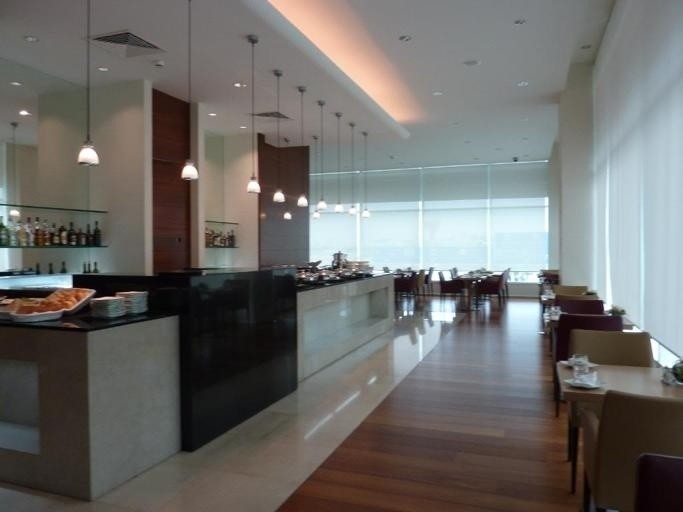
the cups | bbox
[572,354,589,381]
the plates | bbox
[90,296,126,320]
[561,359,600,370]
[117,292,148,315]
[564,378,606,389]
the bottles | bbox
[86,223,94,246]
[36,261,99,274]
[78,227,88,246]
[0,215,50,248]
[93,220,101,246]
[68,222,78,246]
[205,227,235,248]
[58,226,68,245]
[50,222,61,245]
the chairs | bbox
[539,268,682,512]
[382,267,510,311]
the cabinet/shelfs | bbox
[0,202,109,276]
